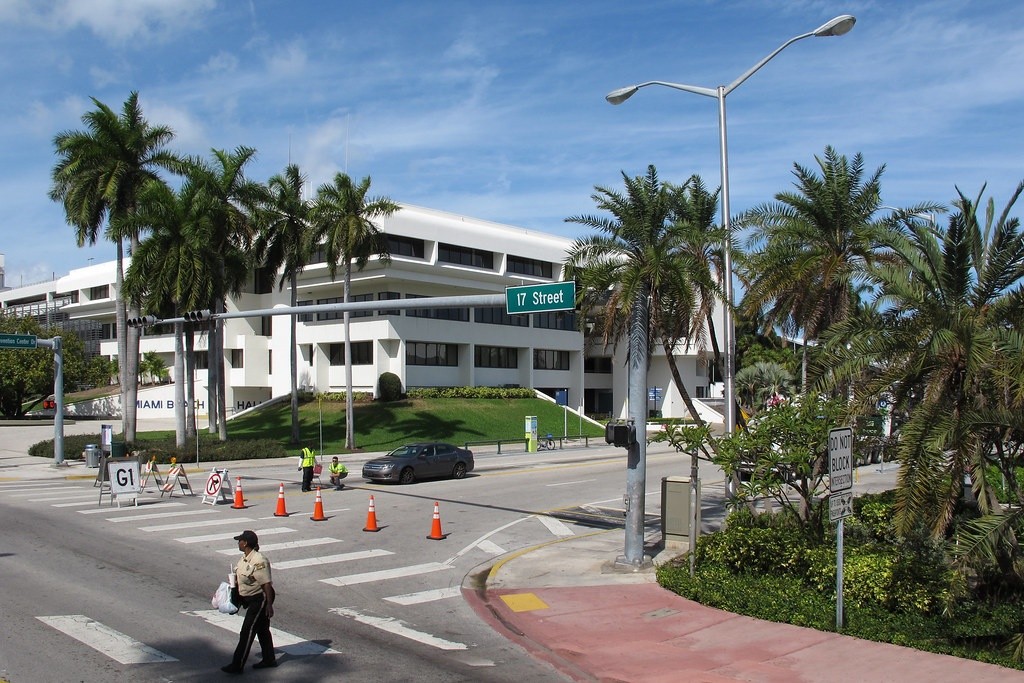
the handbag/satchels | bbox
[211,582,238,613]
[314,465,321,473]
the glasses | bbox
[333,460,338,462]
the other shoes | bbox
[340,484,344,489]
[333,485,341,490]
[252,658,278,669]
[302,487,311,492]
[221,662,244,674]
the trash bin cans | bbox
[85,444,98,468]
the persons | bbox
[220,530,279,675]
[297,442,318,492]
[329,457,349,490]
[767,391,781,408]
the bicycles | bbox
[537,430,556,451]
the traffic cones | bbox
[274,482,289,517]
[310,486,328,521]
[231,476,248,509]
[425,501,447,540]
[362,494,381,532]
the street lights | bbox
[605,11,859,486]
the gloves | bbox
[298,467,302,471]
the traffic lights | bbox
[126,314,156,329]
[183,310,210,323]
[603,422,629,446]
[43,401,55,409]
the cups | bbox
[228,573,236,588]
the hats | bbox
[234,530,258,546]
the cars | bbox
[361,442,474,486]
